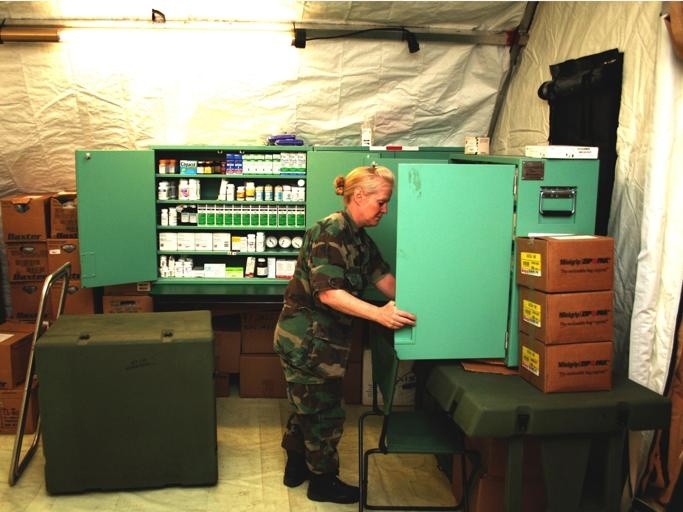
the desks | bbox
[414,360,670,510]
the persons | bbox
[274,166,417,505]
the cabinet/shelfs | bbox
[378,154,600,369]
[78,147,469,301]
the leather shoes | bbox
[307,474,359,504]
[283,451,308,488]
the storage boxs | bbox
[242,315,281,352]
[239,353,289,397]
[48,278,96,319]
[102,284,152,295]
[511,232,617,292]
[11,281,51,320]
[6,242,51,284]
[515,332,616,395]
[215,329,239,375]
[216,371,229,398]
[1,320,46,340]
[0,379,40,436]
[1,332,32,387]
[52,191,79,237]
[2,193,59,242]
[514,290,617,345]
[102,296,153,312]
[48,236,82,278]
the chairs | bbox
[356,348,470,512]
